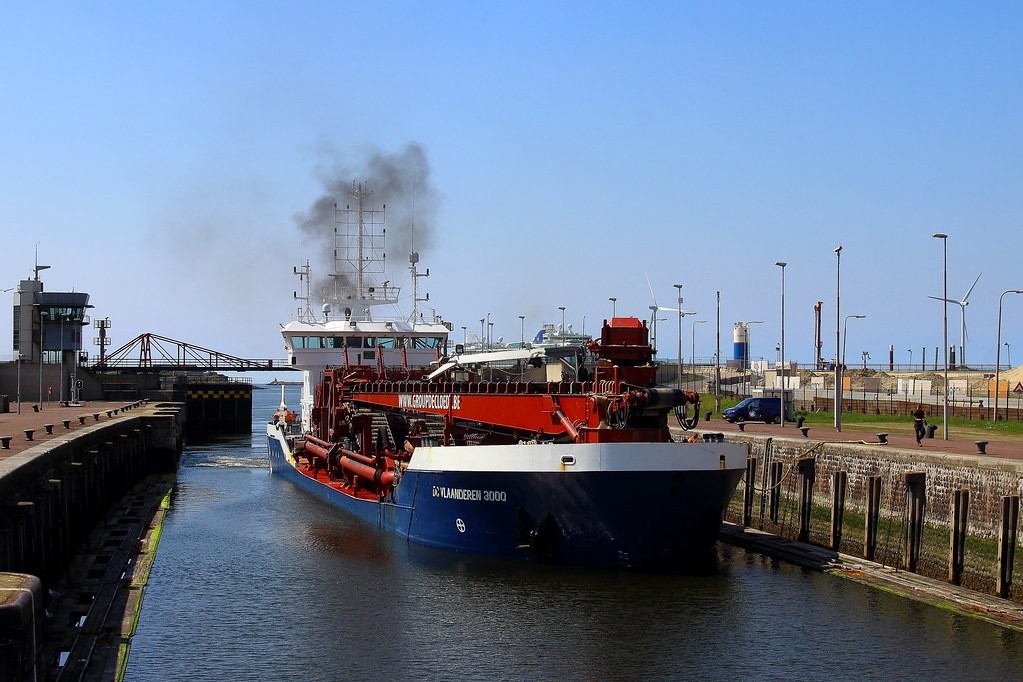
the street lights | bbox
[932,233,948,440]
[518,316,525,350]
[644,272,697,362]
[673,284,683,391]
[481,312,494,353]
[1003,343,1010,369]
[558,306,565,348]
[774,262,788,426]
[692,320,707,393]
[40,312,48,410]
[841,315,866,413]
[928,273,982,371]
[461,326,466,355]
[609,298,618,319]
[833,246,841,432]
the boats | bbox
[267,179,748,560]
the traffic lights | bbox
[75,380,83,389]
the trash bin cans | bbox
[0,395,9,413]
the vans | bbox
[722,396,789,424]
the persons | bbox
[914,404,928,447]
